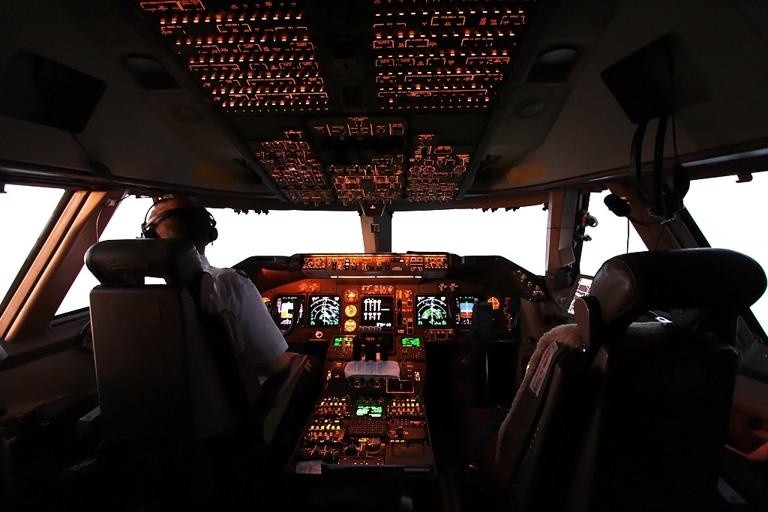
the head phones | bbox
[629,110,689,221]
[143,207,217,242]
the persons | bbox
[147,200,300,377]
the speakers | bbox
[600,32,707,126]
[0,48,106,135]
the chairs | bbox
[489,248,768,509]
[76,237,325,471]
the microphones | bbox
[604,194,669,226]
